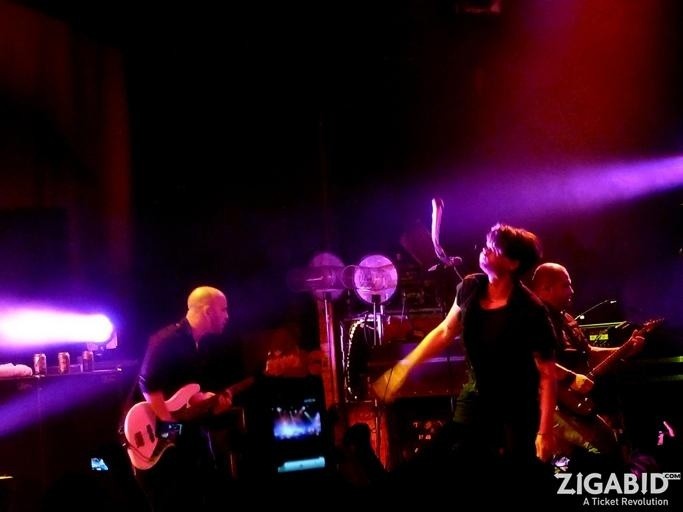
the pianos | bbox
[338,306,475,473]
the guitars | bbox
[123,378,254,471]
[556,318,665,421]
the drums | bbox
[352,255,399,305]
[304,253,346,301]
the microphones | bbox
[427,256,462,272]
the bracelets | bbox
[535,428,555,437]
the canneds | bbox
[59,352,70,374]
[82,350,93,372]
[33,354,48,375]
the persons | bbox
[368,221,563,510]
[117,283,258,510]
[529,262,649,511]
